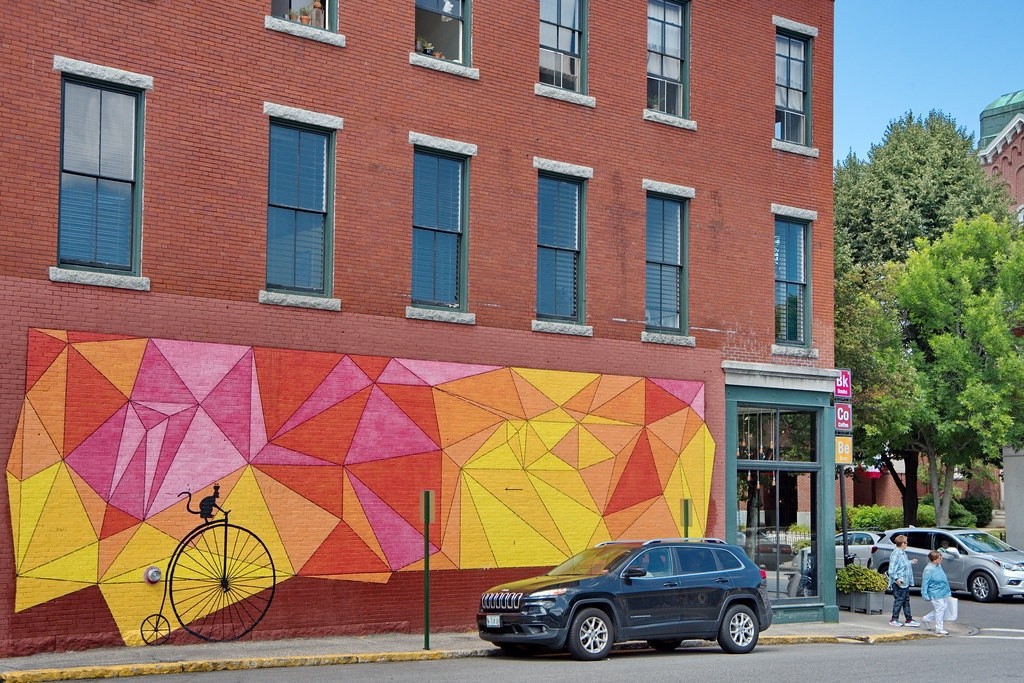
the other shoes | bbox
[904,620,920,626]
[935,629,949,635]
[889,620,904,627]
[921,616,932,631]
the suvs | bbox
[475,537,775,660]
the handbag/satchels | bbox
[943,597,959,621]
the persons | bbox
[937,538,951,553]
[920,550,952,635]
[888,535,921,627]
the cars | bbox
[736,532,795,570]
[870,525,1024,602]
[796,531,887,570]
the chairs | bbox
[647,553,664,572]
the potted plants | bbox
[652,94,658,110]
[836,563,888,615]
[288,9,298,21]
[432,49,443,58]
[423,43,434,55]
[416,33,428,53]
[299,6,312,25]
